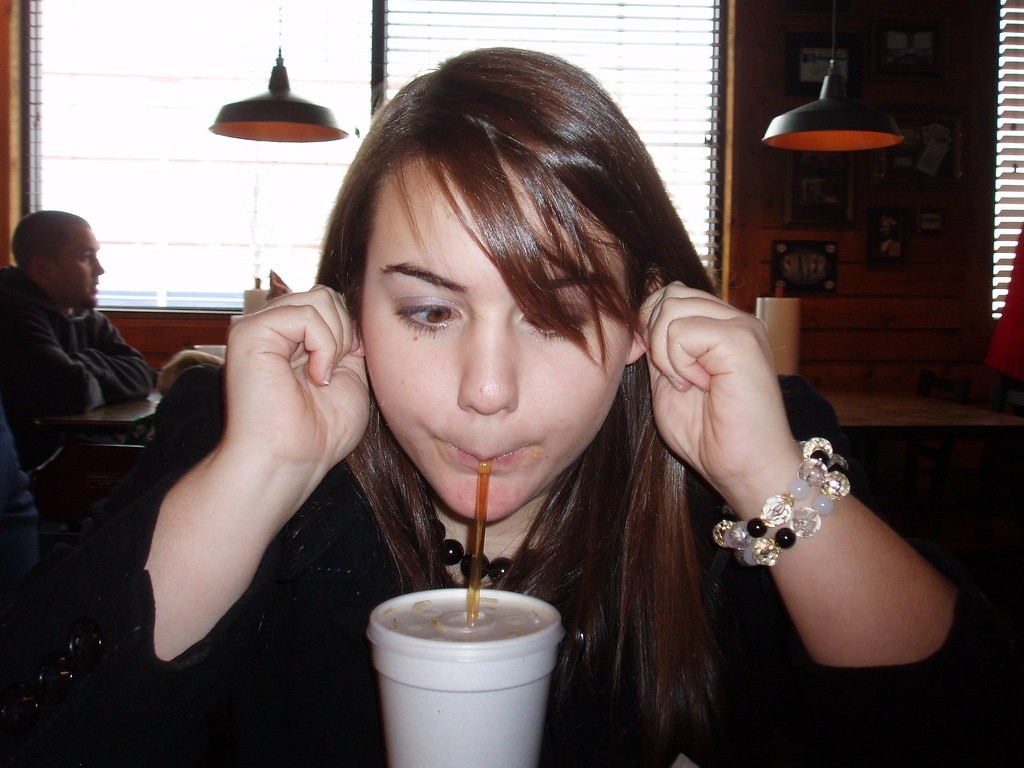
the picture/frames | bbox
[877,105,966,184]
[916,209,946,235]
[785,30,866,100]
[868,12,950,82]
[865,204,914,264]
[780,148,857,227]
[770,238,837,295]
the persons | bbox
[0,48,1024,768]
[0,212,227,709]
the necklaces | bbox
[423,501,545,590]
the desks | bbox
[810,388,1023,526]
[26,386,166,480]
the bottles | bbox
[243,277,268,315]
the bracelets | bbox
[712,437,852,567]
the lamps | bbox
[763,1,906,150]
[209,0,350,142]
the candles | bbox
[755,297,801,377]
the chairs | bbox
[975,373,1024,513]
[863,370,973,507]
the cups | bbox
[195,344,226,361]
[364,587,565,768]
[756,297,800,380]
